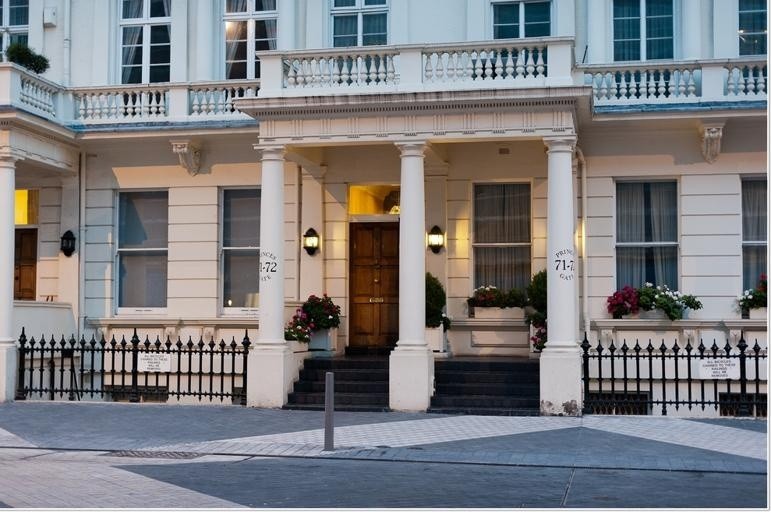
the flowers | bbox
[468,285,528,307]
[287,292,341,336]
[605,282,690,320]
[737,273,769,308]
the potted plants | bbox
[425,271,450,352]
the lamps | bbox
[59,230,76,255]
[303,230,319,255]
[427,225,444,255]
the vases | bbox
[473,305,526,319]
[620,306,669,321]
[308,325,339,351]
[748,306,767,321]
